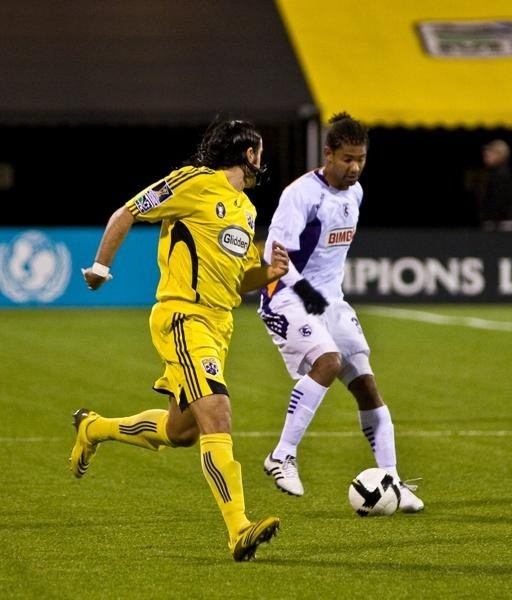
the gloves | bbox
[290,278,330,315]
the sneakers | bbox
[263,452,304,497]
[227,516,280,562]
[399,477,425,512]
[68,407,101,479]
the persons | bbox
[472,140,512,305]
[263,112,424,513]
[69,118,289,562]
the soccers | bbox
[348,468,401,517]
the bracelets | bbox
[92,262,112,281]
